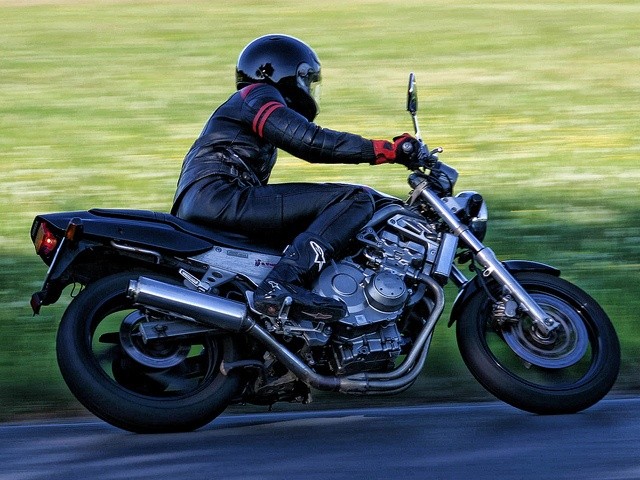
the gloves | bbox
[371,132,420,166]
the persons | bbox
[170,34,420,321]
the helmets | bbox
[235,34,322,122]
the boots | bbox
[253,231,347,323]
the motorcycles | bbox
[30,74,620,433]
